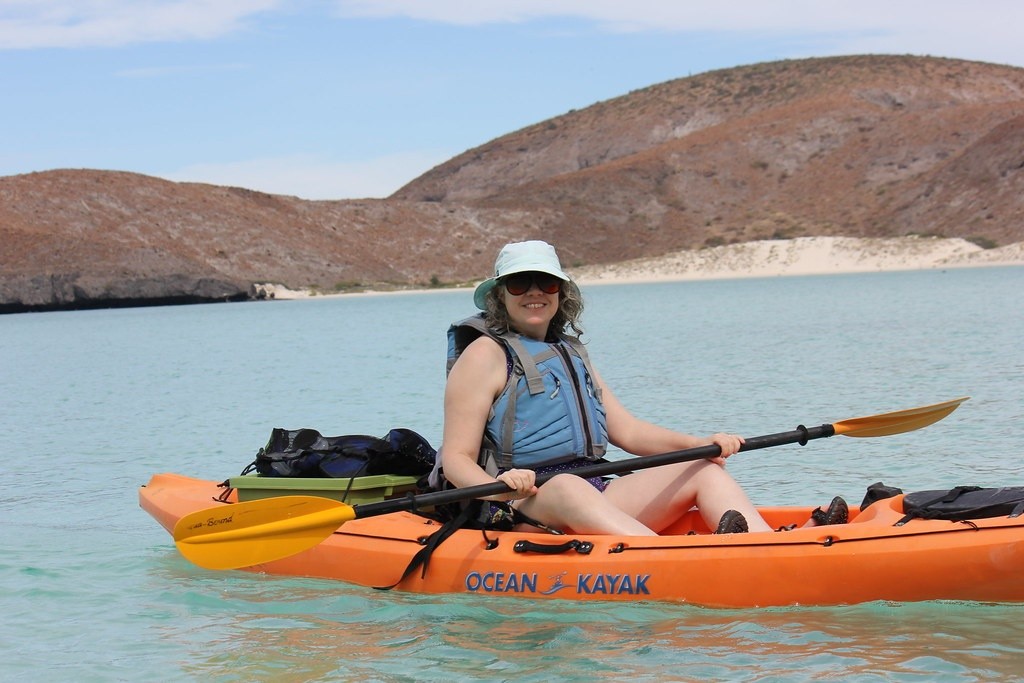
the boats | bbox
[135,463,1023,611]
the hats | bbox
[474,240,571,310]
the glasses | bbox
[499,271,563,296]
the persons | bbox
[442,239,850,538]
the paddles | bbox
[172,395,975,571]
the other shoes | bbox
[685,509,748,535]
[774,496,848,532]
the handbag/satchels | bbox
[212,428,436,504]
